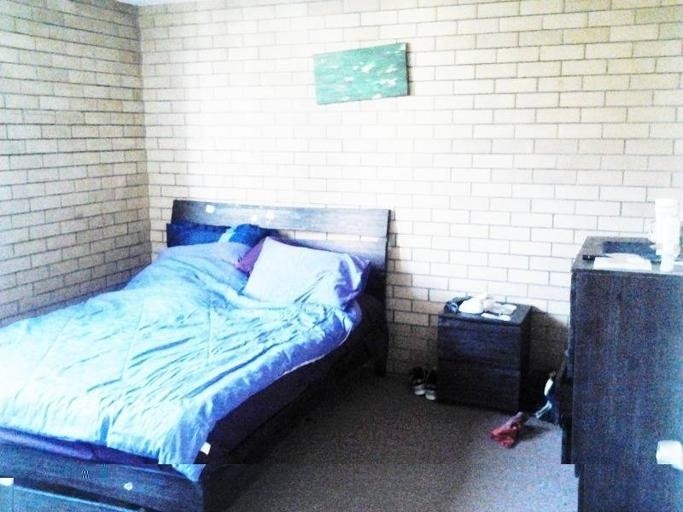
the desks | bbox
[436,302,531,414]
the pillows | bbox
[161,218,372,316]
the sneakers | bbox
[413,366,437,400]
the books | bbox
[601,239,662,265]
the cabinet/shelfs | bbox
[571,236,683,512]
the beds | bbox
[0,201,390,511]
